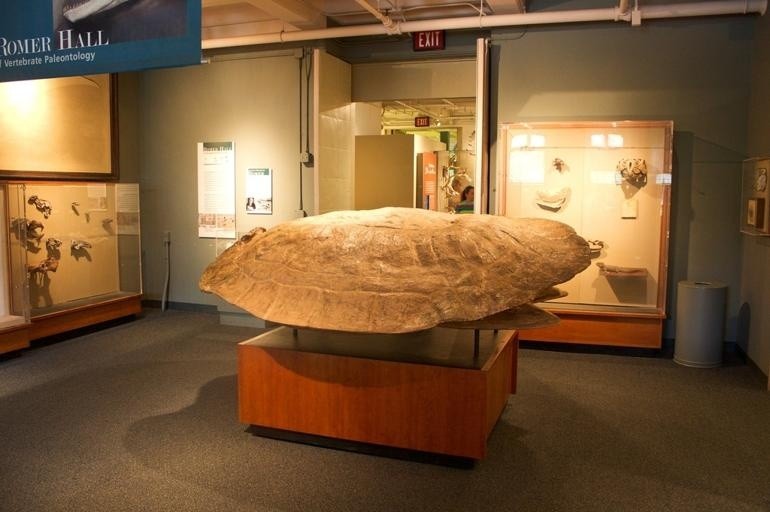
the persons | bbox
[455,186,474,213]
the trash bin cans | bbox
[671,281,728,370]
